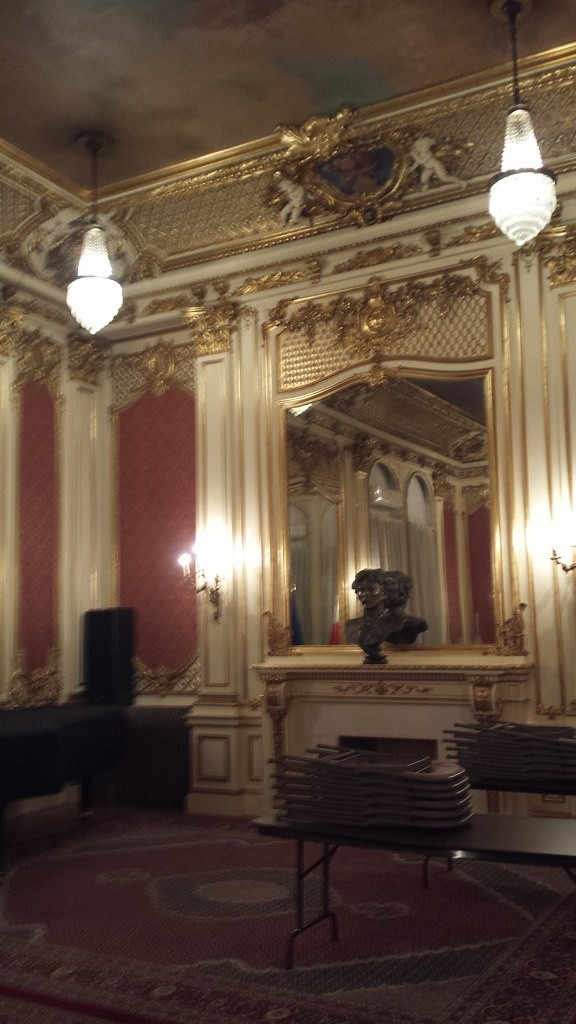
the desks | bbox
[469,777,576,797]
[251,813,576,974]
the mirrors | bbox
[261,363,529,656]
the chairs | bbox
[268,718,576,835]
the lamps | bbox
[178,542,223,623]
[550,545,576,574]
[65,130,125,336]
[486,0,559,248]
